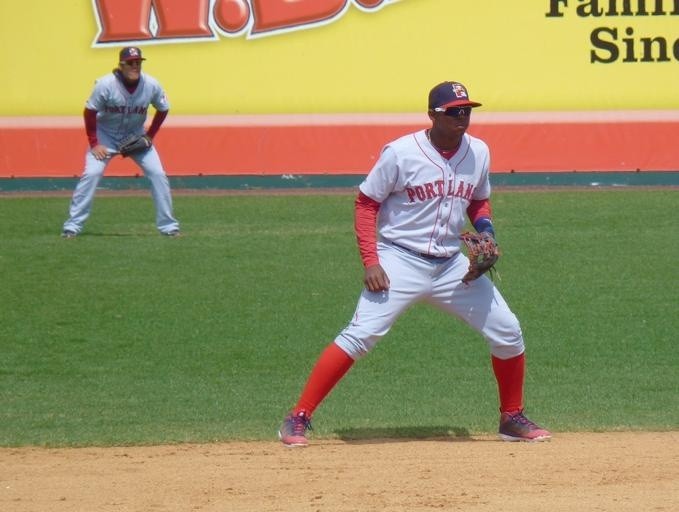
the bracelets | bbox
[474,217,496,240]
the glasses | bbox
[434,108,474,117]
[119,61,143,65]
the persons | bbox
[59,46,184,240]
[277,81,552,449]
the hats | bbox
[119,46,146,62]
[427,80,482,110]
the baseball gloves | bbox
[121,133,152,158]
[459,230,498,283]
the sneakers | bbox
[277,408,313,448]
[165,232,182,238]
[60,229,78,240]
[498,406,552,442]
